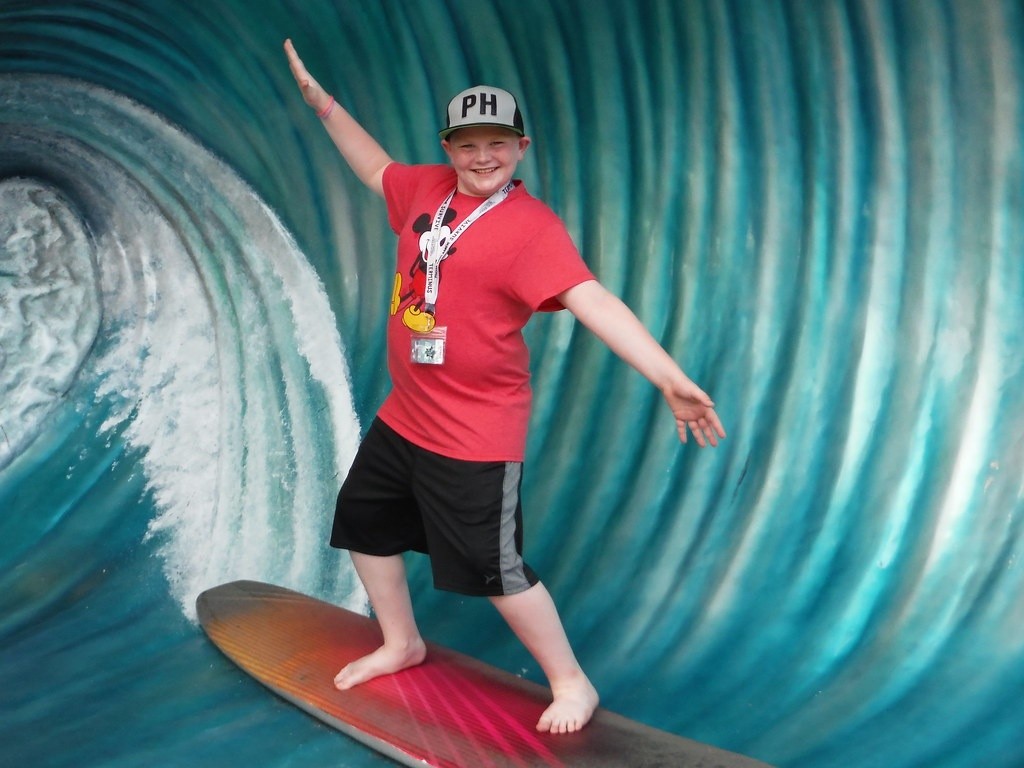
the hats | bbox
[436,84,526,141]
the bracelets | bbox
[316,95,335,119]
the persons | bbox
[281,34,727,735]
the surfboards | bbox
[197,579,773,768]
